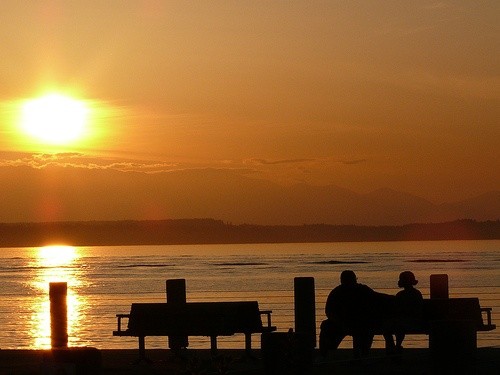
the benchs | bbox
[319,297,497,358]
[113,301,276,358]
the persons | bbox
[382,270,423,347]
[322,270,375,347]
